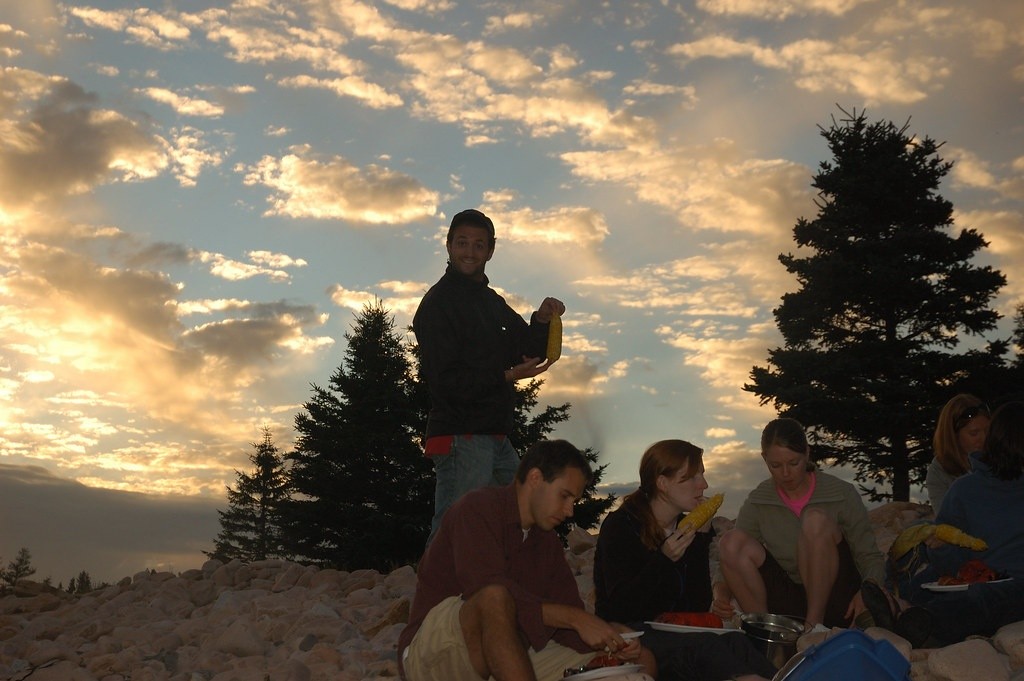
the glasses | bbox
[954,403,990,432]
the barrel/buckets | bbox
[739,612,814,669]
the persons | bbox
[396,438,658,681]
[593,394,1024,680]
[412,209,564,534]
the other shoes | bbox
[861,577,905,632]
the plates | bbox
[921,577,1013,592]
[645,622,740,636]
[562,665,646,681]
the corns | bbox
[677,493,725,534]
[935,523,988,551]
[546,312,562,362]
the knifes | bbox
[619,632,644,639]
